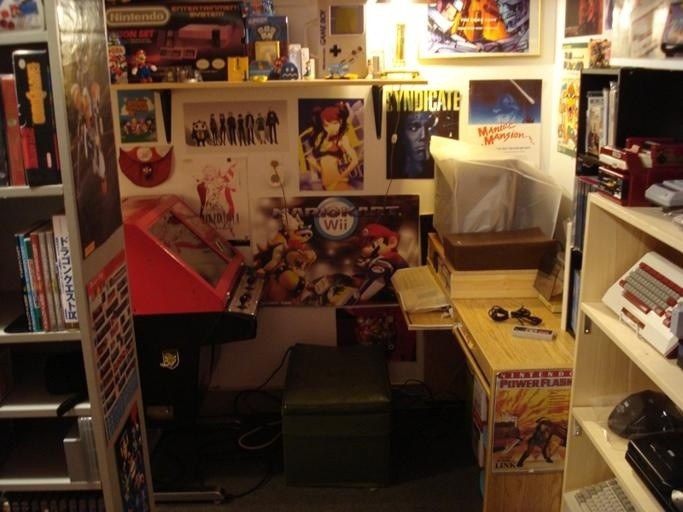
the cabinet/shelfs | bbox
[563,64,682,341]
[557,192,683,510]
[0,0,160,511]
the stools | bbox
[277,342,394,489]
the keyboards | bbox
[391,266,450,313]
[565,478,635,512]
[601,251,683,356]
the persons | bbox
[305,103,358,190]
[358,223,408,303]
[209,107,280,147]
[395,111,439,177]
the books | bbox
[63,417,100,483]
[0,0,80,331]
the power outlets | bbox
[264,151,283,187]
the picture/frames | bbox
[167,91,294,165]
[414,0,542,61]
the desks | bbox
[404,233,578,512]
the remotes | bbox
[512,326,553,340]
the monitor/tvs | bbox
[149,211,228,289]
